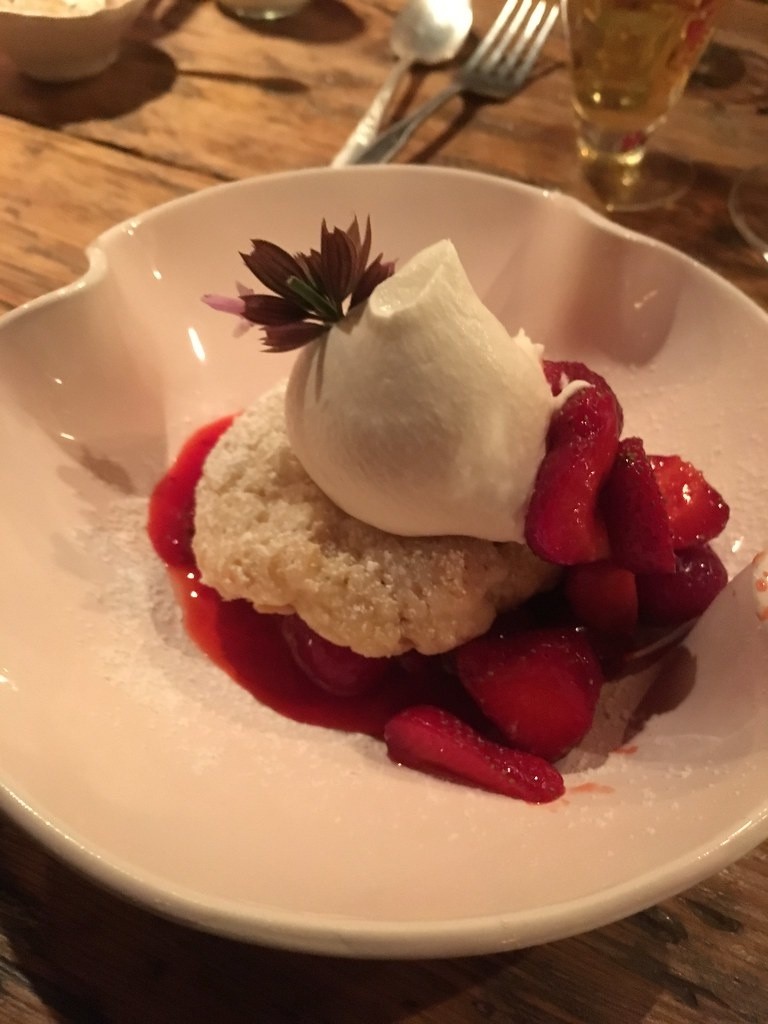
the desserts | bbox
[184,213,734,806]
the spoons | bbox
[328,0,473,166]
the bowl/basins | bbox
[0,0,148,83]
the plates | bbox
[0,167,768,960]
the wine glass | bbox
[516,0,723,214]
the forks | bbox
[353,0,558,166]
